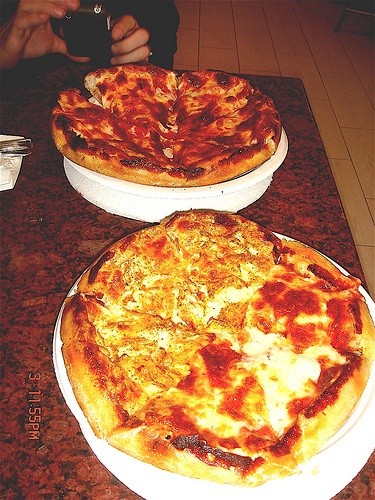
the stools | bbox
[333,1,375,33]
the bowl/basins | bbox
[63,85,289,222]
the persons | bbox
[1,0,179,88]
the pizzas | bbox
[51,64,282,187]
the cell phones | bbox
[63,4,110,58]
